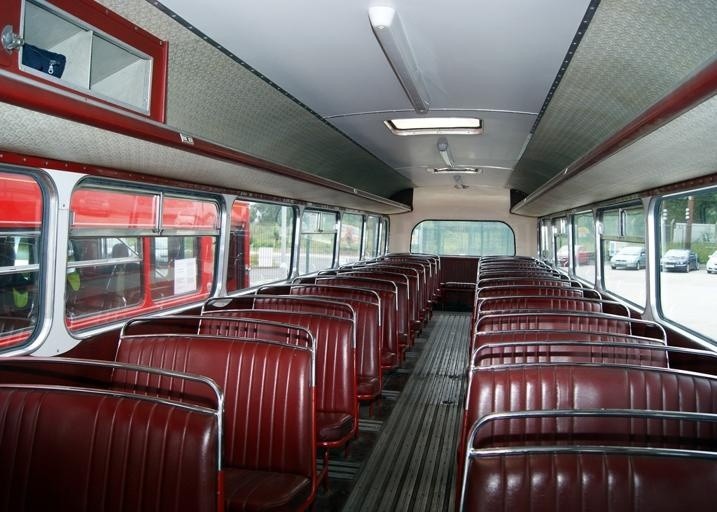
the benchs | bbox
[0,252,441,512]
[459,255,716,510]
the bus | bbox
[0,169,252,353]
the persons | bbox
[0,236,37,329]
[102,242,142,307]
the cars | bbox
[705,250,717,274]
[609,246,646,270]
[551,244,588,267]
[659,248,699,274]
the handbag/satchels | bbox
[22,43,66,78]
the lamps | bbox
[368,7,431,114]
[437,143,455,167]
